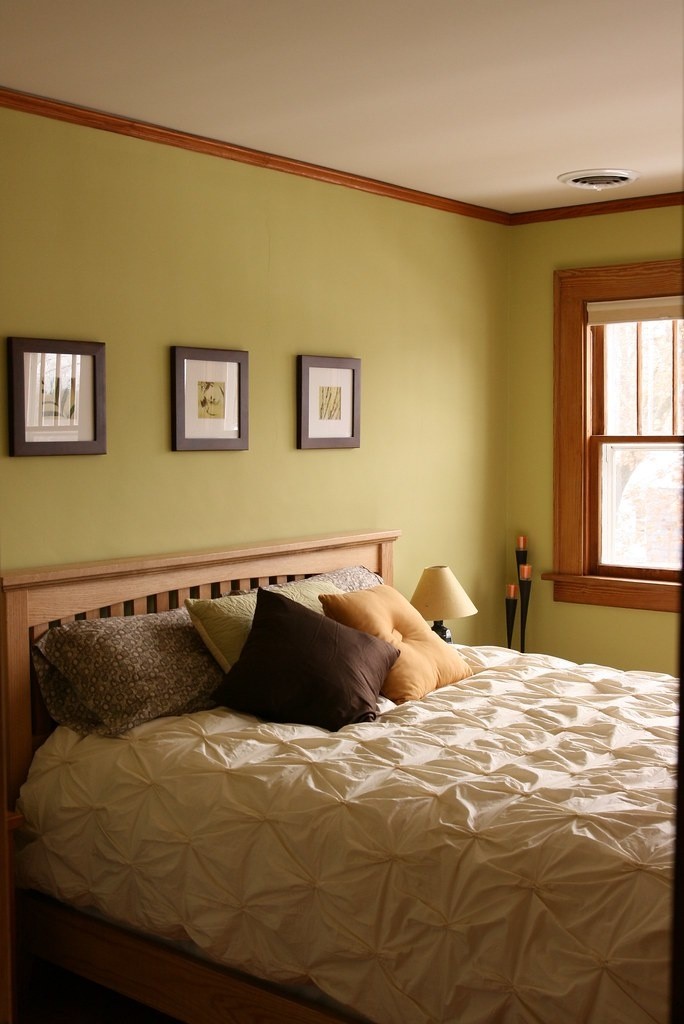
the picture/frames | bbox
[6,336,106,456]
[296,355,361,449]
[170,346,249,452]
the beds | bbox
[0,530,678,1024]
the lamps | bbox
[410,566,478,643]
[505,535,532,653]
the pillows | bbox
[318,587,475,705]
[31,607,225,737]
[183,566,383,675]
[210,586,401,732]
